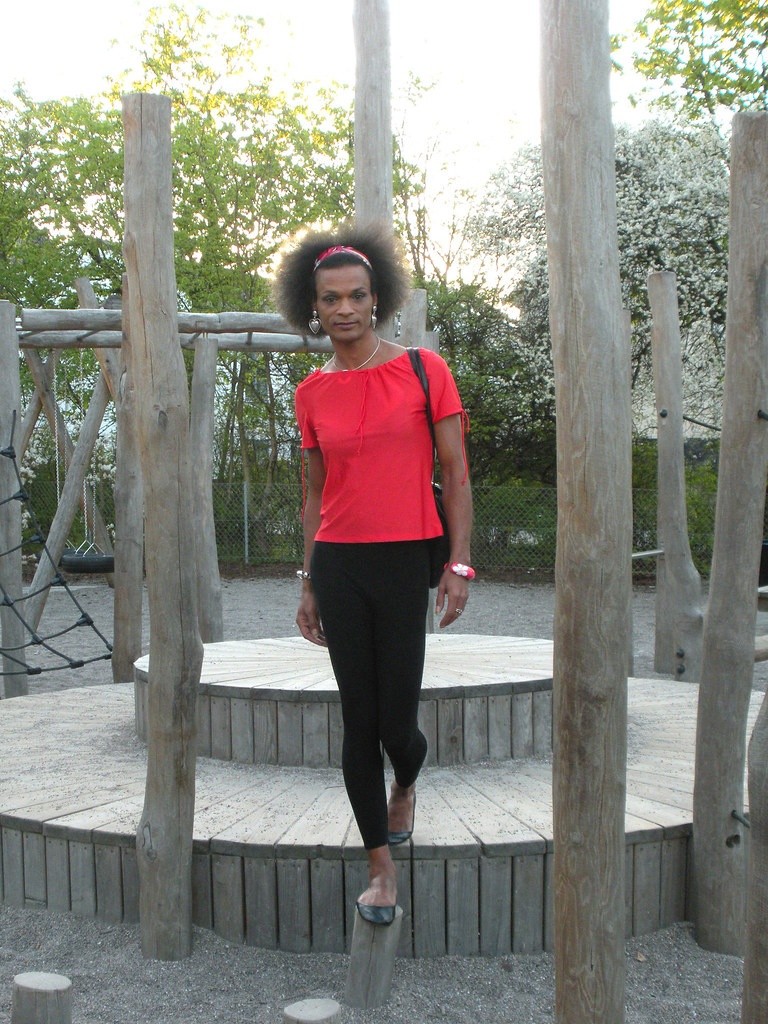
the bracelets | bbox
[444,561,475,580]
[296,570,311,581]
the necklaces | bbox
[333,331,380,371]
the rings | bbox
[455,608,463,613]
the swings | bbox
[37,348,114,575]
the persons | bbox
[273,219,473,926]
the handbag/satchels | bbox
[407,344,452,589]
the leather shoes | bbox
[386,790,417,847]
[355,893,398,927]
